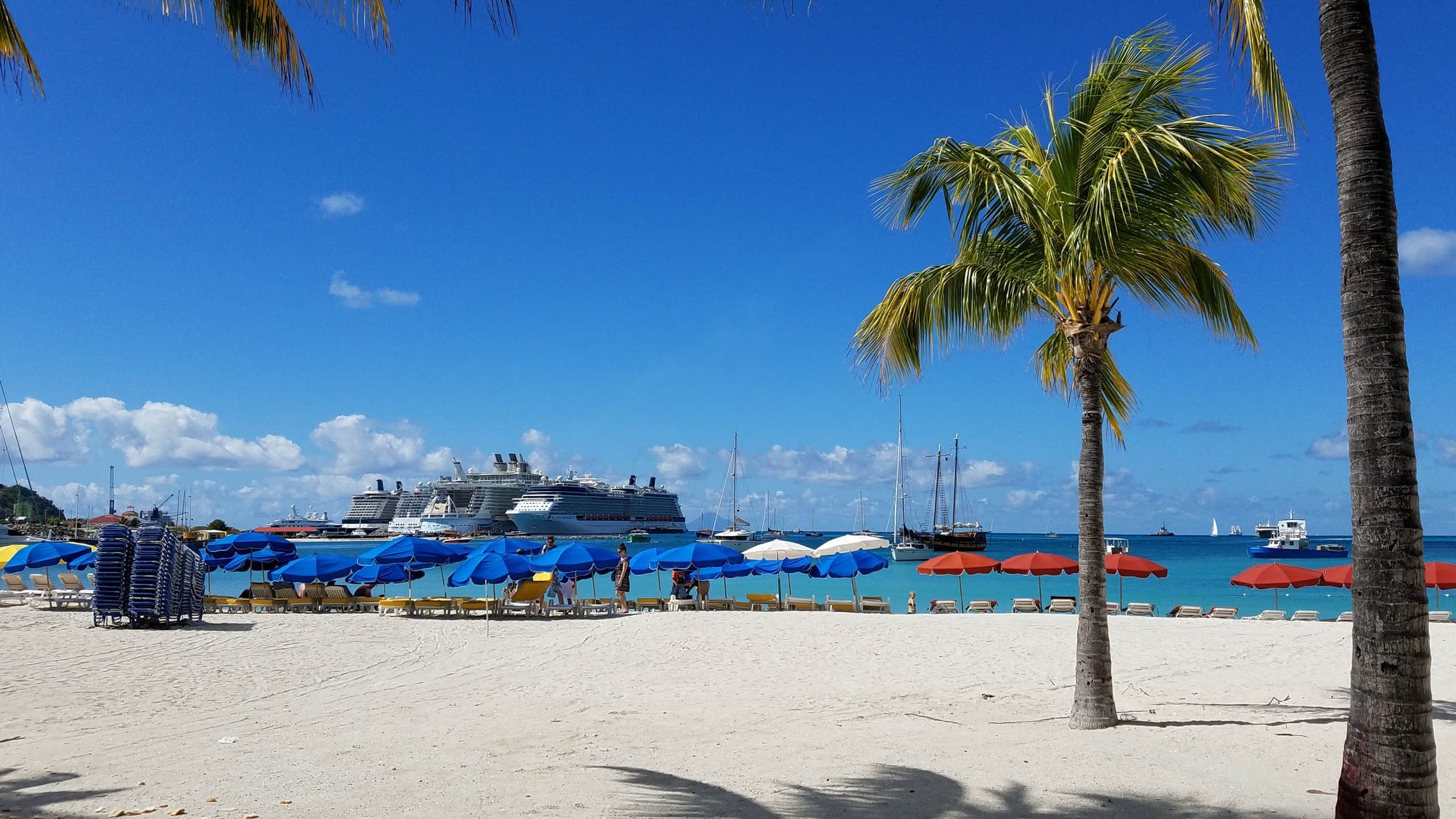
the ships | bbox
[419,452,553,538]
[505,464,690,534]
[387,481,435,535]
[341,479,407,538]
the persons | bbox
[298,581,377,597]
[615,542,629,613]
[672,570,710,600]
[510,536,575,612]
[907,591,916,613]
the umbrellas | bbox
[447,537,543,616]
[688,563,762,599]
[1317,564,1352,589]
[1424,561,1456,610]
[1001,552,1079,612]
[917,550,1001,613]
[629,547,668,605]
[1105,553,1168,615]
[200,532,364,611]
[1230,560,1322,610]
[0,540,97,608]
[647,542,744,609]
[529,540,620,604]
[742,534,889,612]
[345,535,475,615]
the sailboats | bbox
[805,508,824,537]
[850,490,886,539]
[1210,517,1218,537]
[898,431,994,551]
[889,392,936,560]
[696,509,715,538]
[758,489,790,539]
[695,430,766,553]
[68,484,99,545]
[1044,509,1059,537]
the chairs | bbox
[1241,608,1287,622]
[1044,595,1077,614]
[1337,610,1352,622]
[1165,605,1204,619]
[1206,604,1241,619]
[1429,610,1453,623]
[0,520,890,631]
[1291,610,1320,621]
[1123,602,1159,617]
[928,599,960,614]
[1107,601,1121,615]
[1010,598,1040,615]
[966,597,999,614]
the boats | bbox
[1254,517,1279,539]
[647,516,674,522]
[1103,538,1129,558]
[628,527,651,543]
[1146,521,1175,536]
[1245,507,1350,559]
[266,504,343,528]
[792,526,805,534]
[435,524,472,544]
[587,514,624,521]
[1228,525,1243,536]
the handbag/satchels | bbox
[611,567,631,581]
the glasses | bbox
[547,540,553,543]
[618,549,624,553]
